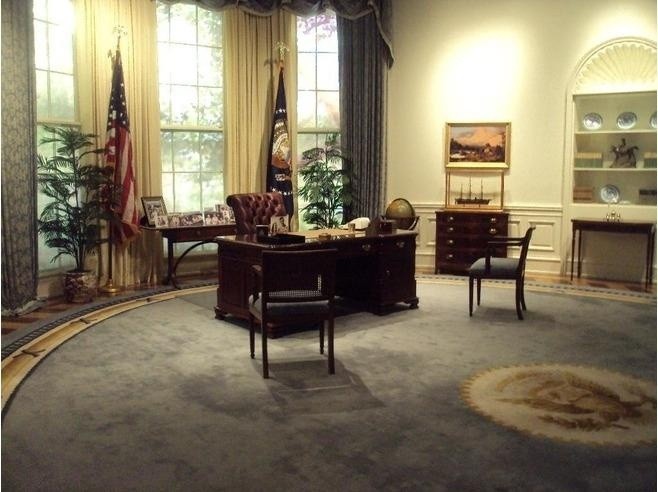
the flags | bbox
[104,50,143,252]
[265,67,294,231]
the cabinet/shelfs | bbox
[434,210,509,274]
[564,87,656,208]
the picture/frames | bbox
[444,120,511,169]
[140,195,169,226]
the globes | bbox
[384,197,419,231]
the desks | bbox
[568,216,657,293]
[140,224,271,292]
[211,220,420,339]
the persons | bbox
[150,204,235,227]
[278,216,288,232]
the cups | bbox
[256,224,268,239]
[348,224,355,233]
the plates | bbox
[616,111,637,130]
[582,112,603,131]
[600,184,620,203]
[648,111,657,129]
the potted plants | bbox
[34,124,123,306]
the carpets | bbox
[2,275,655,491]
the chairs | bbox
[249,247,337,377]
[226,191,286,234]
[466,224,535,321]
